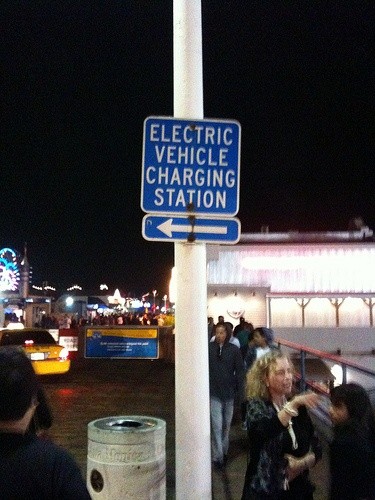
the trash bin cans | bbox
[87,414,167,500]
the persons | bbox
[327,383,375,500]
[239,346,323,500]
[208,322,245,471]
[205,314,279,431]
[0,346,91,500]
[2,309,175,330]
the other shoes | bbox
[213,455,228,471]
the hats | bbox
[261,326,275,344]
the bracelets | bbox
[283,402,299,417]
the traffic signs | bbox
[139,115,242,246]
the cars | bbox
[0,322,73,376]
